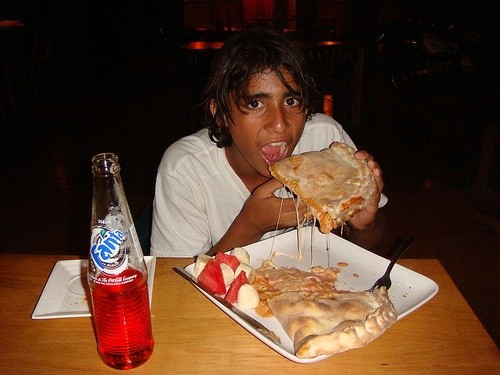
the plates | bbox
[184,225,440,365]
[31,254,156,320]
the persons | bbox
[150,30,389,258]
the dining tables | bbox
[0,253,500,375]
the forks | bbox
[366,234,420,293]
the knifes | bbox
[172,267,282,346]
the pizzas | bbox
[250,268,337,317]
[266,285,397,359]
[268,141,376,233]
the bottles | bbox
[87,153,154,370]
